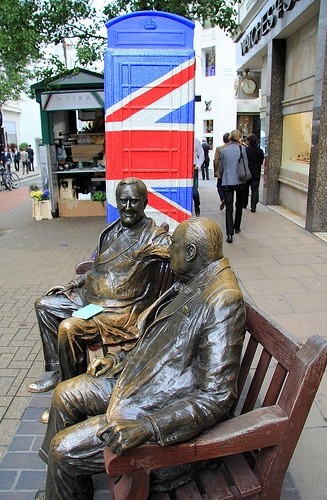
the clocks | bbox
[240,77,257,95]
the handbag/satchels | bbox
[237,145,252,180]
[26,160,31,166]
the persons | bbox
[25,145,35,171]
[26,176,174,425]
[192,137,206,218]
[200,139,211,181]
[1,147,12,180]
[213,133,230,210]
[31,217,247,500]
[218,128,252,242]
[20,148,29,175]
[242,133,265,213]
[12,147,21,171]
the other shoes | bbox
[226,236,233,243]
[220,201,226,210]
[251,208,256,212]
[235,230,241,234]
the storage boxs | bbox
[56,198,107,217]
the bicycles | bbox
[0,163,20,191]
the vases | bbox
[32,200,53,220]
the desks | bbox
[54,166,105,173]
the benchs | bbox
[87,222,327,500]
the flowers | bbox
[29,184,50,200]
